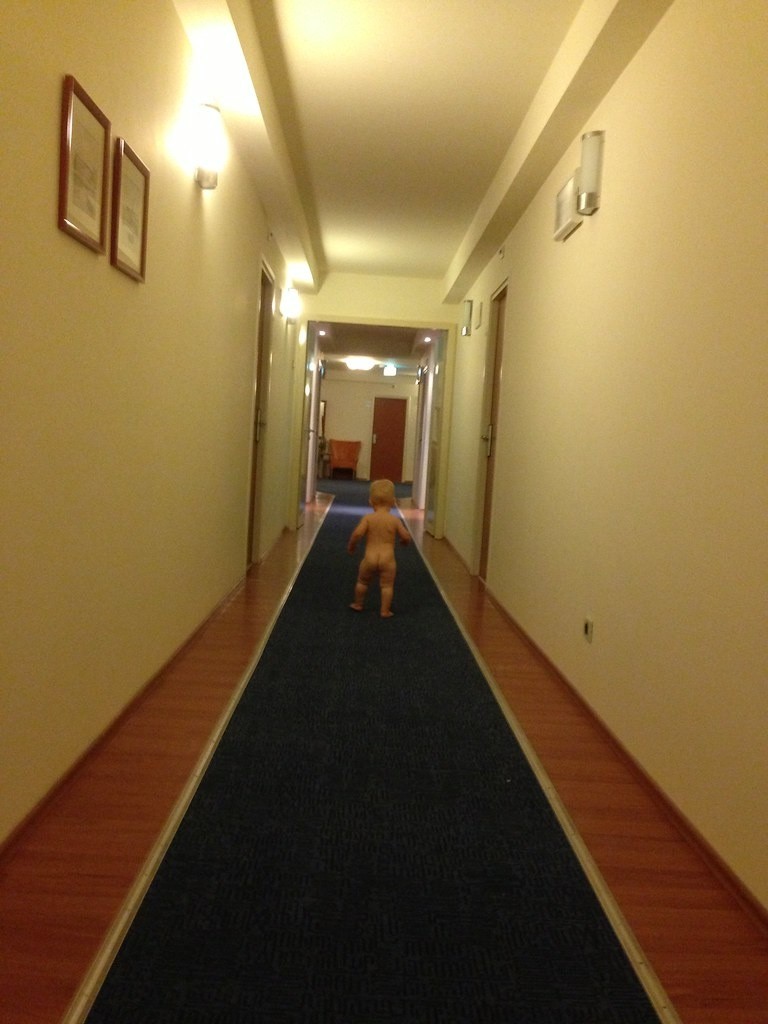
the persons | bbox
[347,479,412,617]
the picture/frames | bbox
[49,73,113,256]
[106,137,154,282]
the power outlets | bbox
[583,616,593,645]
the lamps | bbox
[192,101,224,187]
[576,127,604,216]
[460,298,473,338]
[284,287,299,324]
